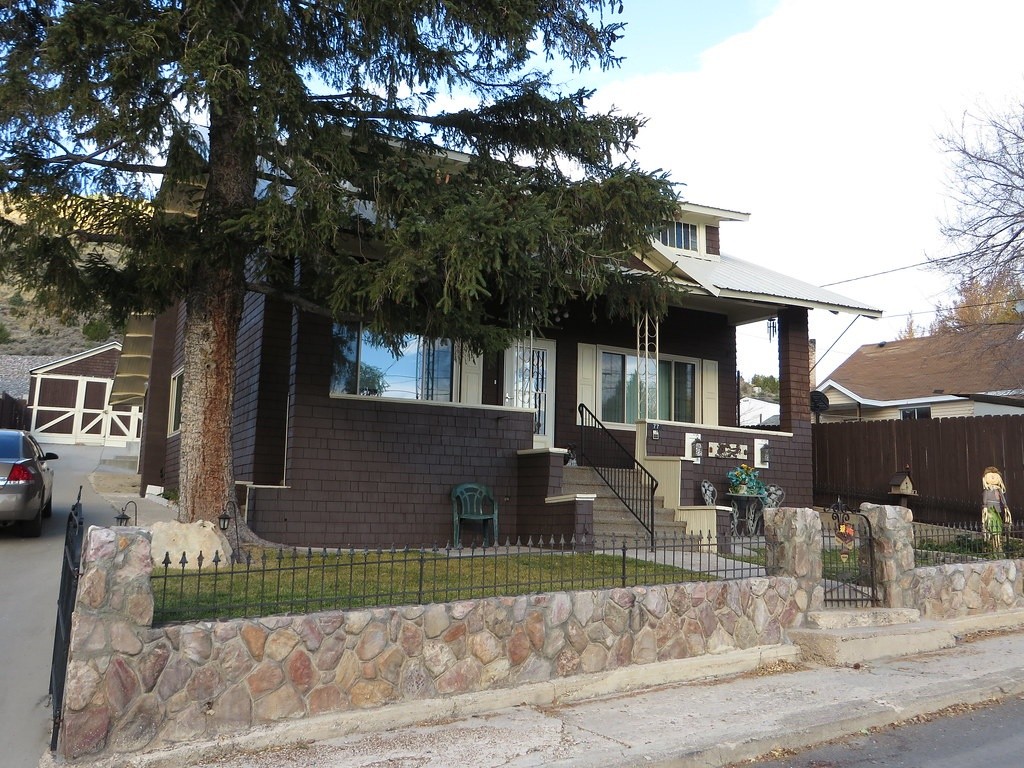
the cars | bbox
[0,429,59,537]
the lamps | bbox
[218,501,244,565]
[113,501,137,526]
[692,437,704,458]
[760,443,773,463]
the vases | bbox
[735,485,748,494]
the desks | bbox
[727,491,768,538]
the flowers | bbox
[727,464,767,495]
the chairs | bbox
[450,482,499,547]
[701,479,742,539]
[753,483,786,536]
